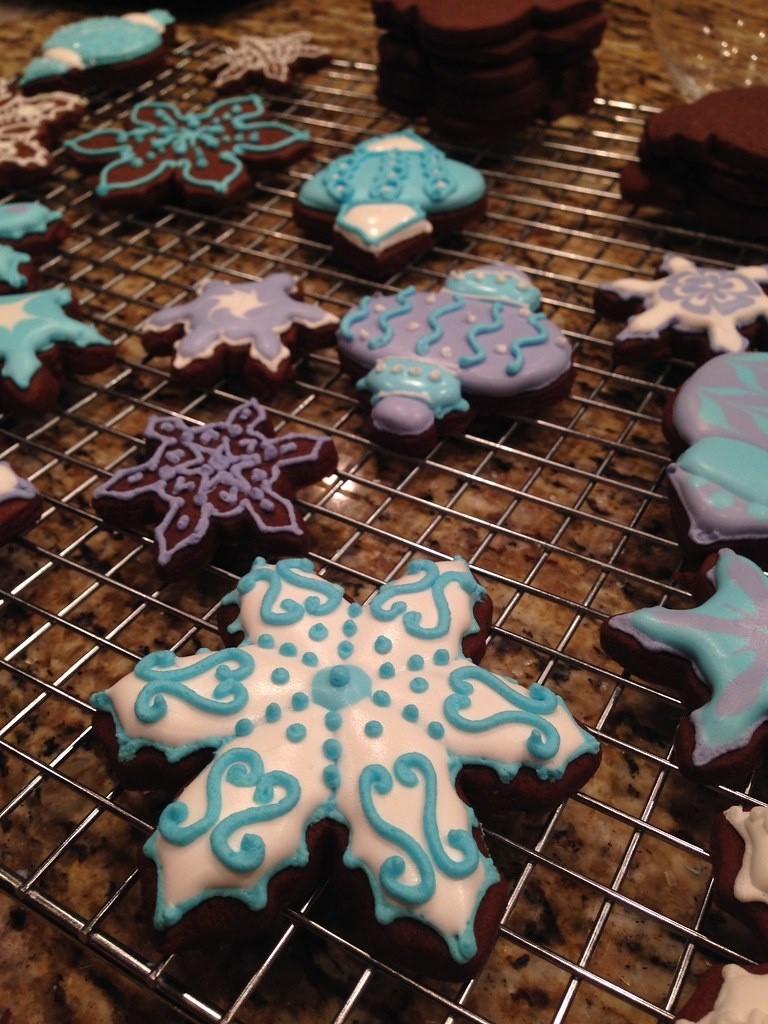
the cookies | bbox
[0,0,768,1024]
